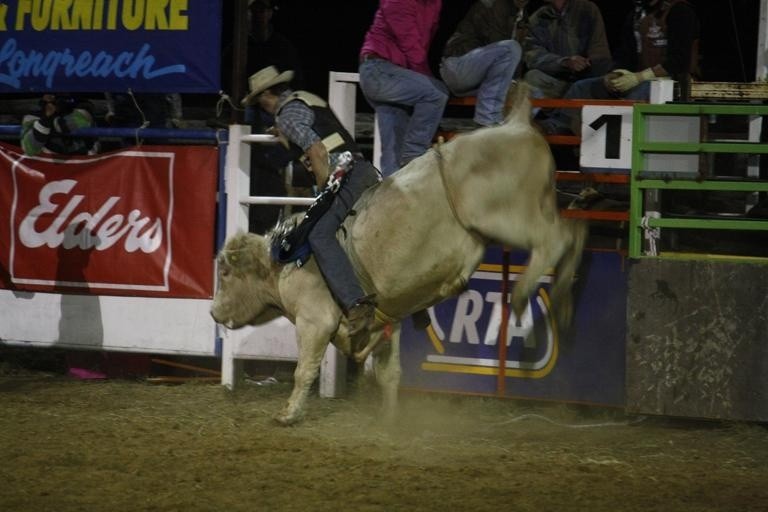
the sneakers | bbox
[347,294,374,337]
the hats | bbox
[240,64,294,105]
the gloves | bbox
[605,68,656,93]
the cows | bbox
[209,77,589,437]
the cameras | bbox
[55,96,76,107]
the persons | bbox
[242,65,378,362]
[19,92,94,158]
[360,1,669,180]
